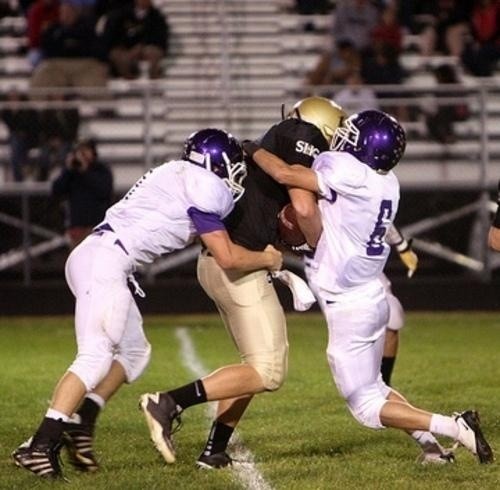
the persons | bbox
[12,127,284,486]
[51,139,119,256]
[241,108,494,475]
[485,178,500,253]
[285,0,500,149]
[383,223,420,388]
[136,94,349,474]
[0,2,171,187]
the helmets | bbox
[286,96,349,144]
[181,129,248,203]
[330,110,406,174]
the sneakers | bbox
[195,450,232,469]
[453,409,493,465]
[421,453,455,465]
[139,391,183,464]
[63,423,99,473]
[11,436,64,481]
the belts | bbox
[115,240,129,255]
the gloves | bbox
[395,239,417,278]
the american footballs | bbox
[277,204,305,247]
